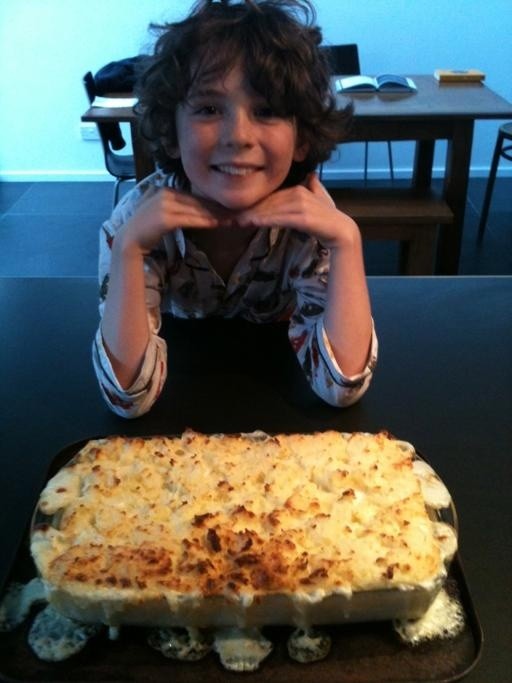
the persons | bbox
[91,0,380,419]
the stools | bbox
[475,122,512,243]
[322,178,456,277]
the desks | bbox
[2,272,510,679]
[82,71,512,273]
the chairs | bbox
[315,43,395,182]
[81,69,138,210]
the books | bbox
[334,73,418,93]
[433,68,484,82]
